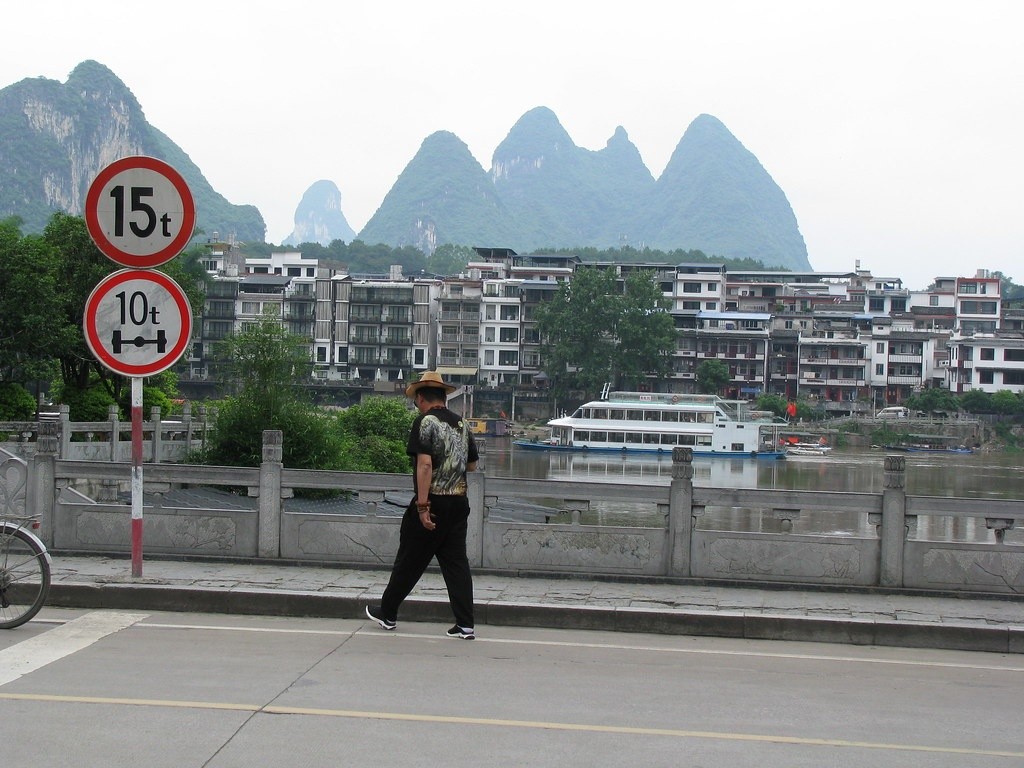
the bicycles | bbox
[0,512,53,628]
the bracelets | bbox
[415,500,430,512]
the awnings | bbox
[435,367,477,375]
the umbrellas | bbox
[311,371,315,378]
[354,368,359,378]
[487,371,504,387]
[377,368,381,381]
[291,365,296,376]
[397,369,403,379]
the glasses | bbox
[413,396,419,408]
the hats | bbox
[406,371,457,398]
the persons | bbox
[366,371,479,639]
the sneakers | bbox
[446,625,476,640]
[365,604,398,631]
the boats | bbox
[786,442,833,457]
[512,391,787,460]
[882,432,973,455]
